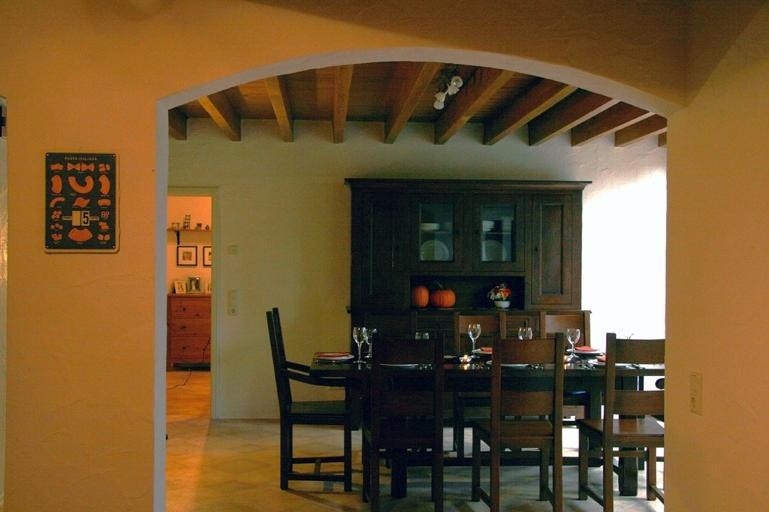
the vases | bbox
[495,299,510,308]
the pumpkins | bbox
[432,282,456,307]
[412,286,431,308]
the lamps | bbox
[431,64,464,110]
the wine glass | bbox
[352,323,580,364]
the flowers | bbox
[486,282,513,301]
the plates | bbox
[420,239,449,261]
[422,214,516,233]
[313,348,631,367]
[479,239,507,262]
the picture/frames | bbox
[176,245,212,266]
[174,275,203,294]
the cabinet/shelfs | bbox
[343,177,592,431]
[166,294,212,371]
